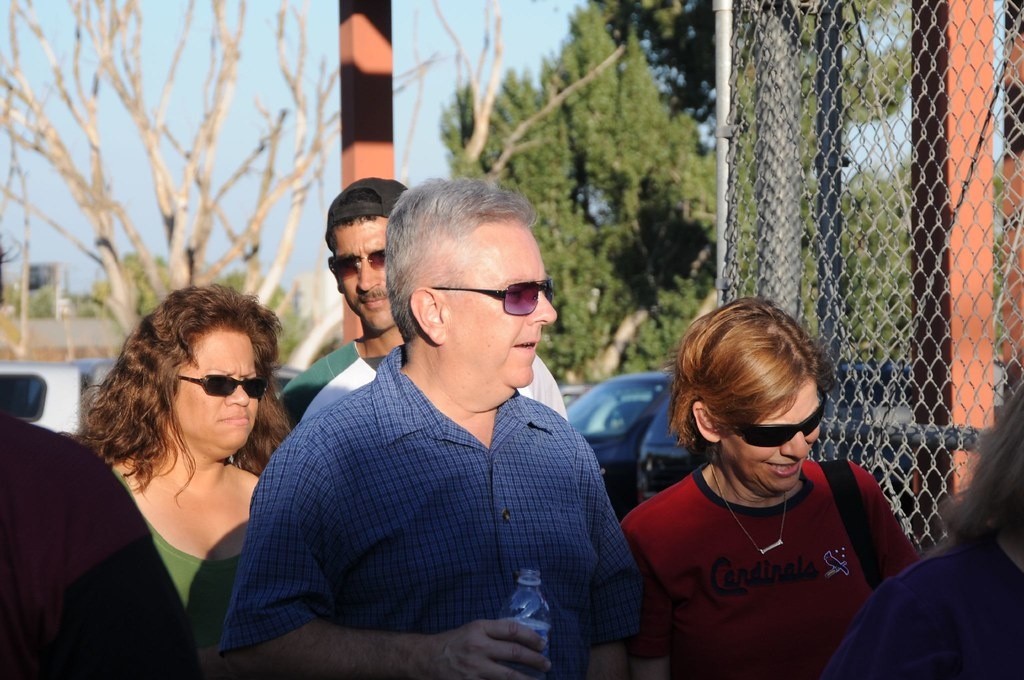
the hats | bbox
[325,177,409,253]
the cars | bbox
[556,356,1024,557]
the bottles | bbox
[496,566,553,680]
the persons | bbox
[620,296,917,680]
[278,177,566,426]
[817,383,1024,680]
[216,179,644,679]
[0,411,205,680]
[59,285,292,680]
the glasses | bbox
[717,386,828,447]
[328,247,387,280]
[178,374,268,401]
[432,275,553,315]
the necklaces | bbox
[708,463,787,554]
